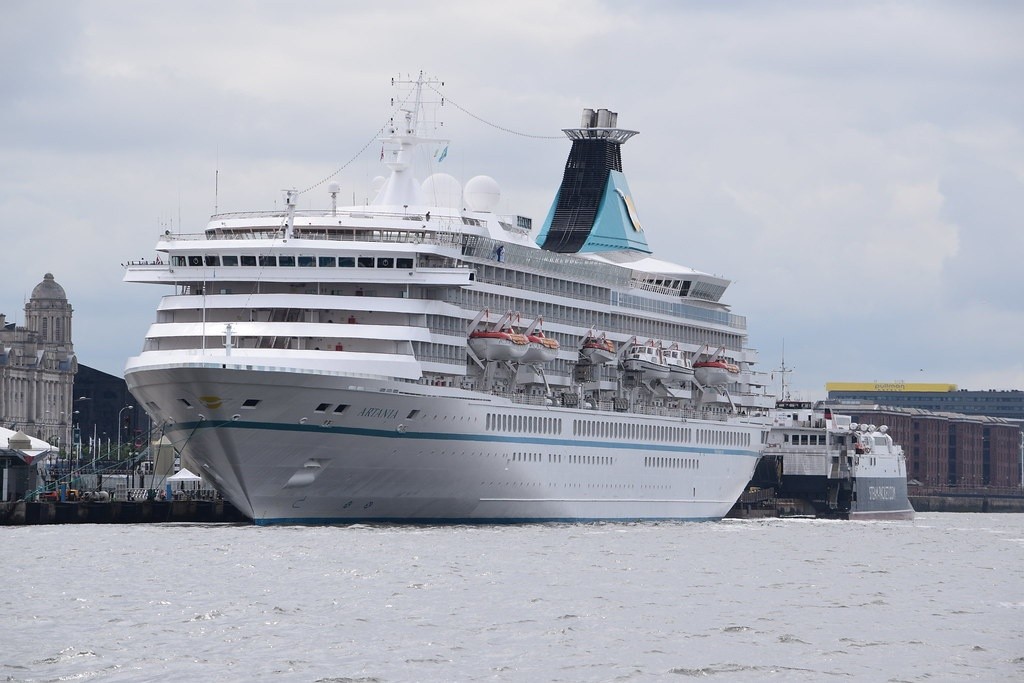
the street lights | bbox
[117,405,134,463]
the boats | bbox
[460,306,741,416]
[0,457,250,526]
[752,404,918,522]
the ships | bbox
[116,70,780,526]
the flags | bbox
[434,146,448,161]
[824,408,832,419]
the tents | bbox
[166,467,202,499]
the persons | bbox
[425,211,431,221]
[497,245,505,261]
